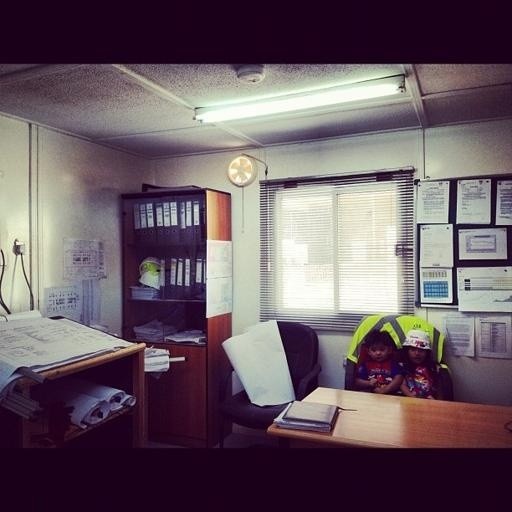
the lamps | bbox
[191,74,407,127]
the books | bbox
[272,400,341,435]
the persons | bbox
[398,329,438,399]
[354,329,403,394]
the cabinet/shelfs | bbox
[0,313,148,452]
[122,188,232,451]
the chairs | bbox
[343,312,457,401]
[213,320,324,452]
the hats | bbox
[402,330,432,350]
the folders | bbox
[160,254,206,299]
[133,197,201,243]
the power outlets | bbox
[13,241,26,256]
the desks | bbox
[265,387,512,451]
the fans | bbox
[226,153,268,236]
